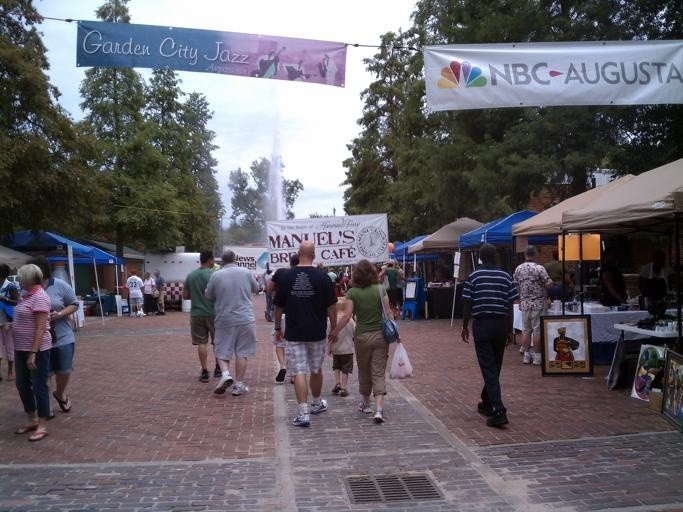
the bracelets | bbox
[58,311,62,319]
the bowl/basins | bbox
[430,283,441,289]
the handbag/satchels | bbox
[3,303,14,322]
[381,318,400,344]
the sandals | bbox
[331,382,342,394]
[341,387,351,397]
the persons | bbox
[0,264,19,383]
[26,255,80,419]
[543,250,569,283]
[459,243,519,426]
[141,272,156,316]
[598,252,627,306]
[554,325,579,362]
[154,269,166,315]
[10,264,53,442]
[125,269,147,317]
[639,249,674,300]
[512,244,555,364]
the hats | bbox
[130,269,138,273]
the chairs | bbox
[401,290,429,321]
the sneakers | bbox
[486,411,509,427]
[310,399,328,414]
[373,409,386,422]
[358,400,373,417]
[519,345,542,366]
[477,401,495,416]
[232,382,249,396]
[292,413,311,427]
[200,372,209,383]
[214,374,234,395]
[214,367,222,377]
[275,367,287,382]
[7,370,15,382]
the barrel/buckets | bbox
[182,299,192,313]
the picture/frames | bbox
[538,313,594,379]
[659,348,682,437]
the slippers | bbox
[29,428,49,442]
[15,421,38,434]
[47,390,73,420]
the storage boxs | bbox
[591,342,613,365]
[402,276,424,303]
[645,388,664,412]
[121,305,129,314]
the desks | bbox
[69,300,85,329]
[81,294,114,315]
[423,285,472,319]
[512,302,683,365]
[605,319,678,393]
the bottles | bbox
[655,321,678,333]
[443,281,450,287]
[52,261,68,284]
[551,297,577,313]
